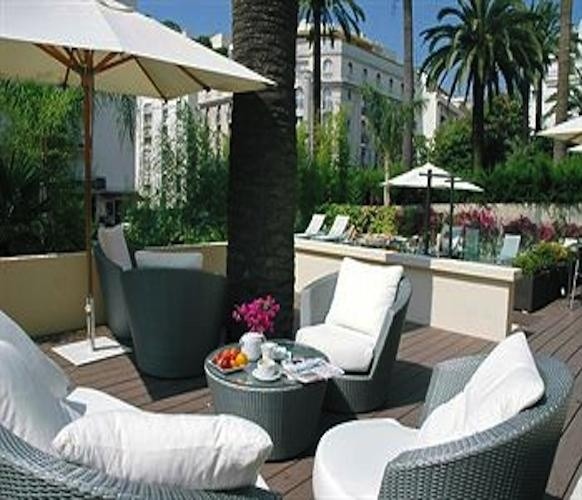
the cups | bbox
[261,340,283,358]
[258,357,278,377]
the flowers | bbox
[233,295,280,330]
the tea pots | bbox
[238,332,261,364]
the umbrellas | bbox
[531,115,582,146]
[380,161,484,192]
[1,1,277,347]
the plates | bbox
[251,369,284,384]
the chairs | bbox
[298,265,412,412]
[0,306,283,500]
[293,214,326,238]
[94,241,132,341]
[314,215,350,240]
[312,355,576,498]
[496,233,521,265]
[119,268,229,379]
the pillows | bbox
[420,332,544,448]
[134,251,204,268]
[324,255,405,340]
[96,224,132,271]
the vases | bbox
[238,332,266,361]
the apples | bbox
[211,342,248,369]
